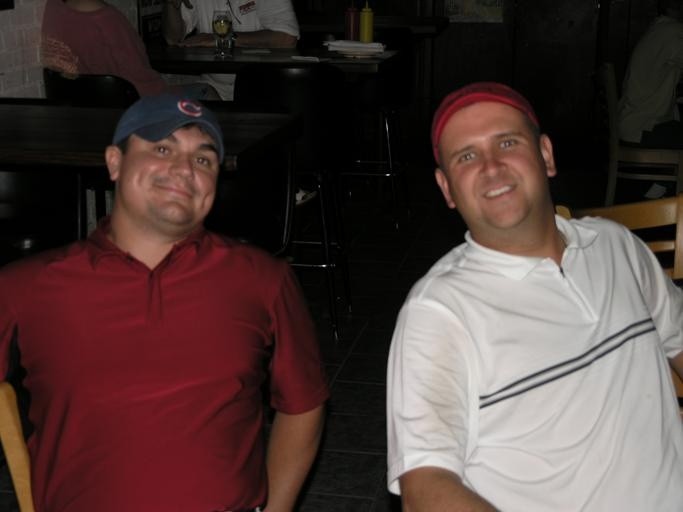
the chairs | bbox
[551,61,682,414]
[234,60,355,344]
[0,354,38,512]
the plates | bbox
[325,41,387,58]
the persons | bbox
[1,92,328,512]
[615,2,681,204]
[383,80,681,512]
[161,0,319,52]
[40,0,319,213]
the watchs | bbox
[227,28,238,49]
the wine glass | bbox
[213,11,233,58]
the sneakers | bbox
[296,187,316,206]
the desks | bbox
[0,17,450,296]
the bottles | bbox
[345,0,374,43]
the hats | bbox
[113,94,226,164]
